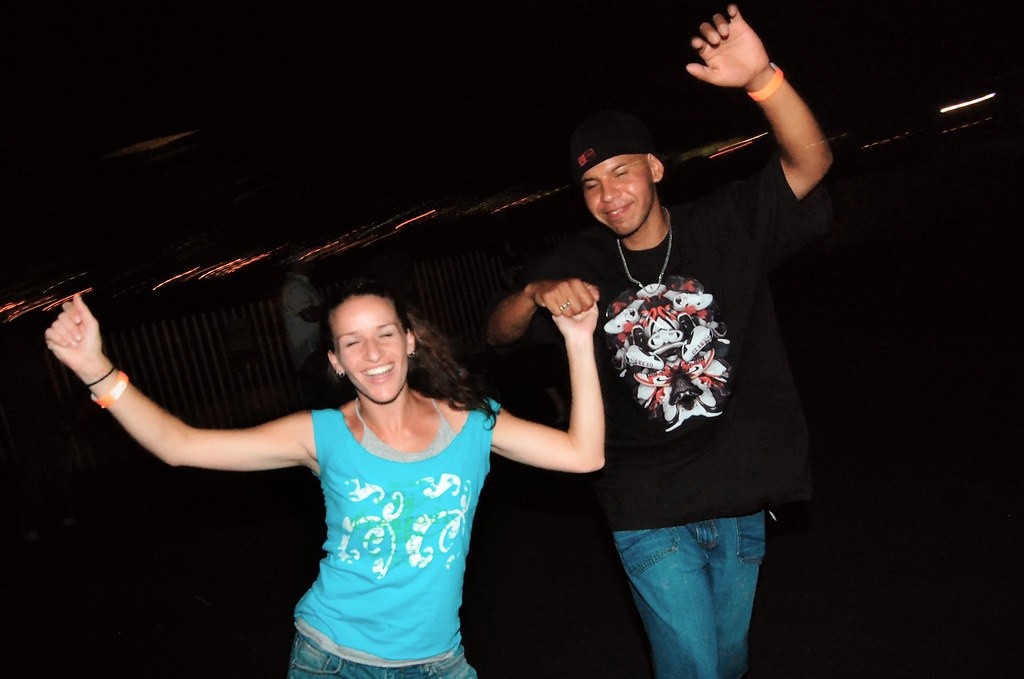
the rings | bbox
[560,302,571,311]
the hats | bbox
[565,110,654,182]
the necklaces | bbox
[616,205,672,295]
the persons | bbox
[485,5,833,679]
[277,224,571,426]
[45,283,606,679]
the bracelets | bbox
[86,366,115,387]
[91,371,128,408]
[747,63,783,101]
